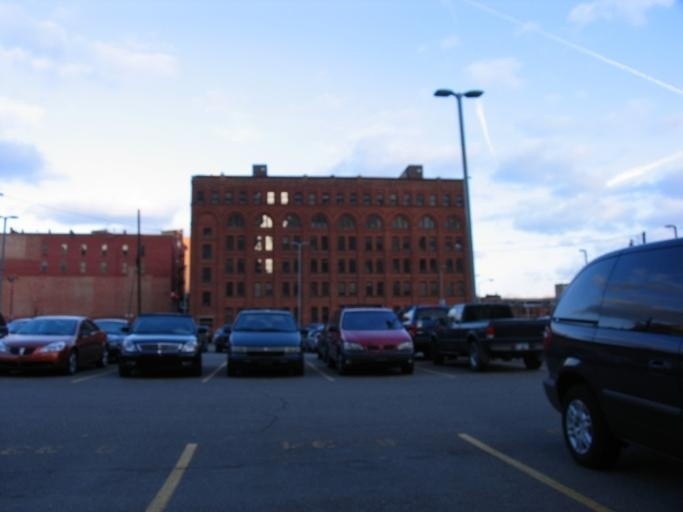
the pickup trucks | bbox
[429,301,551,370]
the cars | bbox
[543,237,682,464]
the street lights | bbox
[433,88,485,301]
[281,239,308,321]
[1,213,19,300]
